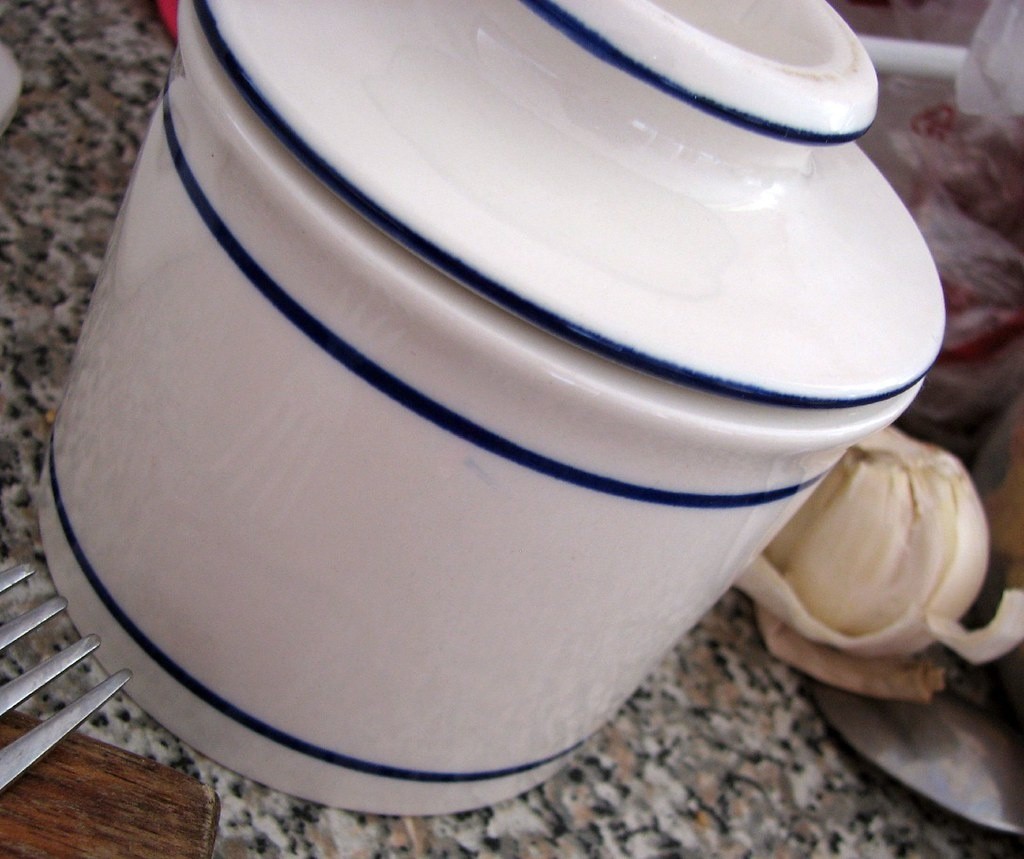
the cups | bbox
[35,0,948,817]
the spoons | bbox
[813,680,1024,837]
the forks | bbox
[0,563,133,794]
